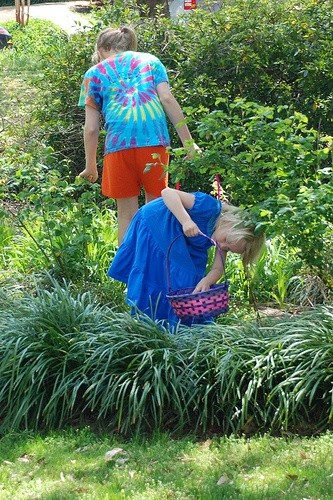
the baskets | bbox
[165,234,229,323]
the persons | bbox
[106,187,265,331]
[77,26,203,249]
